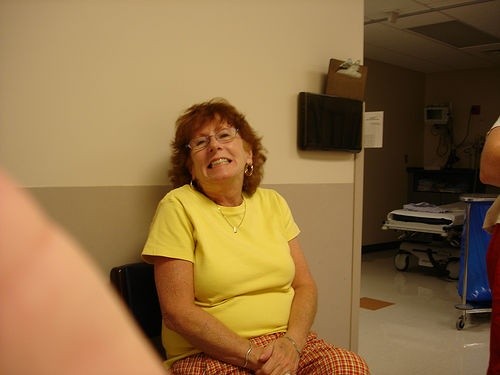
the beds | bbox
[381,199,468,278]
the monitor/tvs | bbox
[296,91,363,151]
[424,107,449,122]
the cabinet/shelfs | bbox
[407,167,476,207]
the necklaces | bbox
[219,196,247,232]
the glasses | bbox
[187,128,240,151]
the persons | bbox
[142,99,372,375]
[0,171,171,375]
[479,119,500,375]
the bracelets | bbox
[243,341,252,368]
[284,336,301,356]
